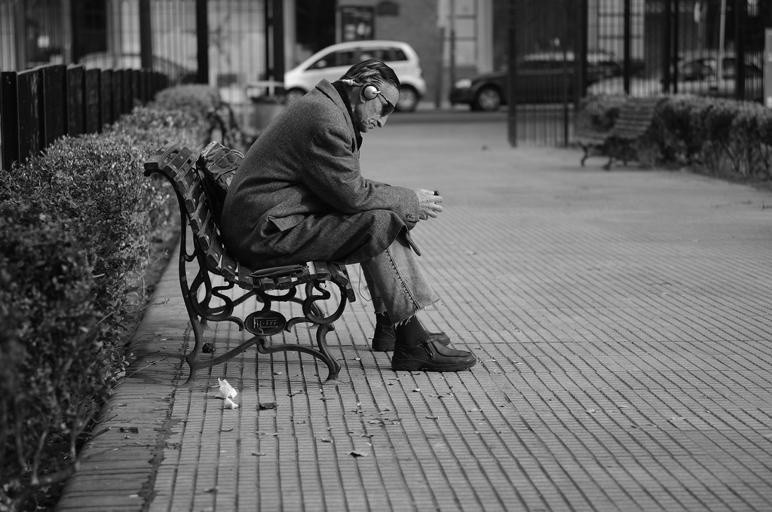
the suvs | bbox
[248,40,429,115]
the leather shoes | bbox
[372,322,450,352]
[392,337,475,371]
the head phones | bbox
[337,77,382,104]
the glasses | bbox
[379,93,396,113]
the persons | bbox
[219,59,477,370]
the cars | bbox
[68,53,198,86]
[448,49,619,111]
[579,50,763,110]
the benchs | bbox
[157,141,355,382]
[574,94,670,171]
[207,100,259,153]
